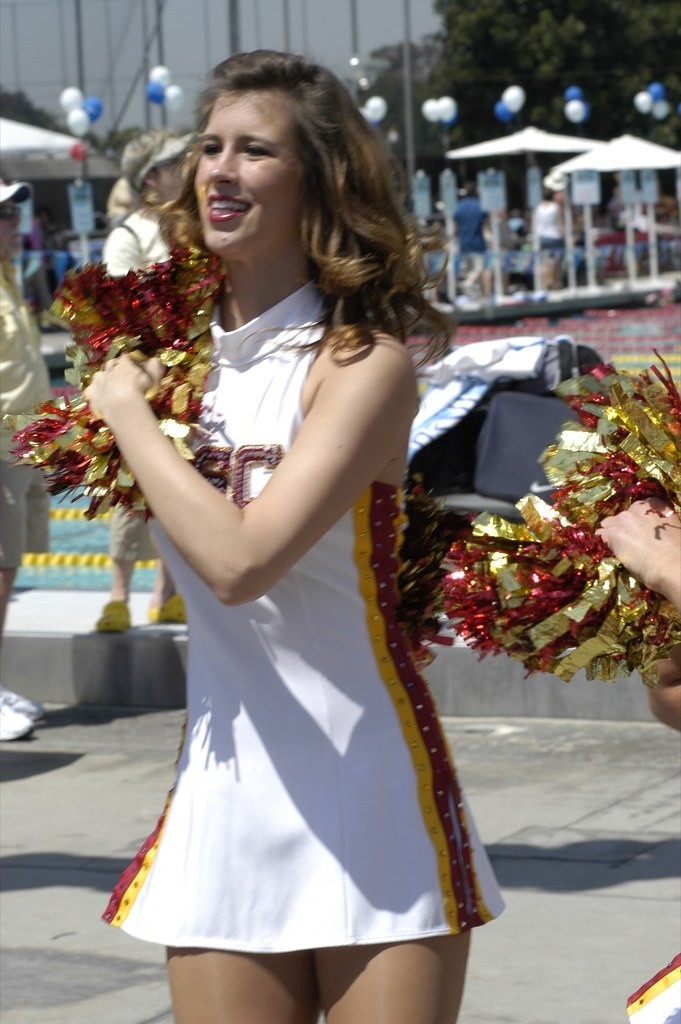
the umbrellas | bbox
[441,123,609,169]
[548,128,680,189]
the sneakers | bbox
[1,700,34,741]
[0,683,40,719]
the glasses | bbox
[1,206,23,221]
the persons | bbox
[95,128,193,635]
[592,490,681,1024]
[506,182,624,251]
[0,177,57,741]
[450,182,493,295]
[85,48,509,1024]
[533,188,565,290]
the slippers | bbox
[100,602,135,632]
[149,595,190,627]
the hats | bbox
[1,173,32,204]
[135,132,188,189]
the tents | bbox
[0,117,124,268]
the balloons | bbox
[163,84,184,102]
[67,108,90,136]
[494,85,525,123]
[359,95,389,130]
[419,97,459,126]
[83,96,103,120]
[634,82,670,119]
[563,86,591,123]
[148,64,172,88]
[145,82,166,104]
[57,86,86,112]
